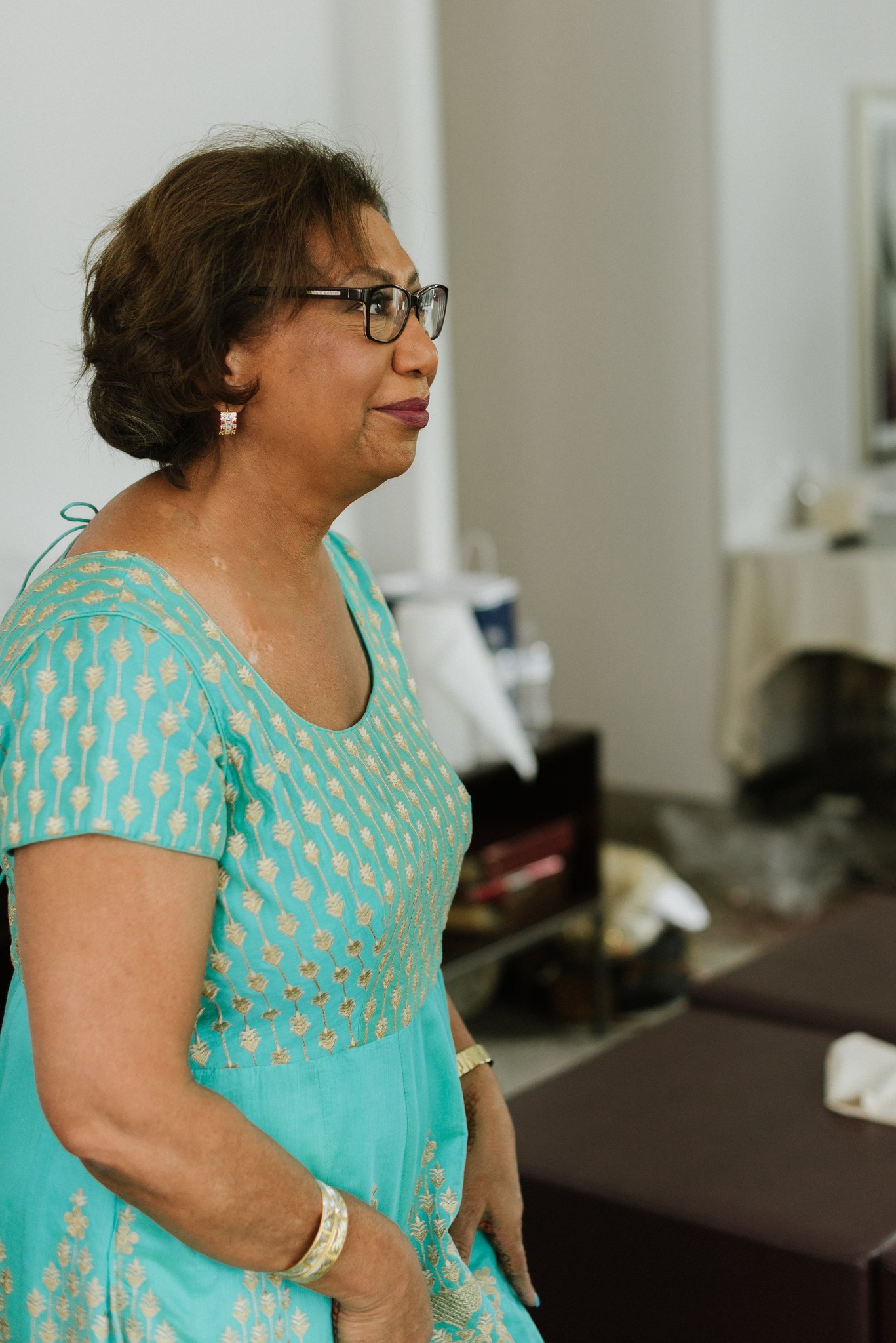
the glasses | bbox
[232,280,449,344]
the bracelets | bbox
[271,1175,351,1287]
[451,1043,494,1080]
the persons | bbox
[0,120,556,1343]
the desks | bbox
[437,715,617,1054]
[506,876,896,1342]
